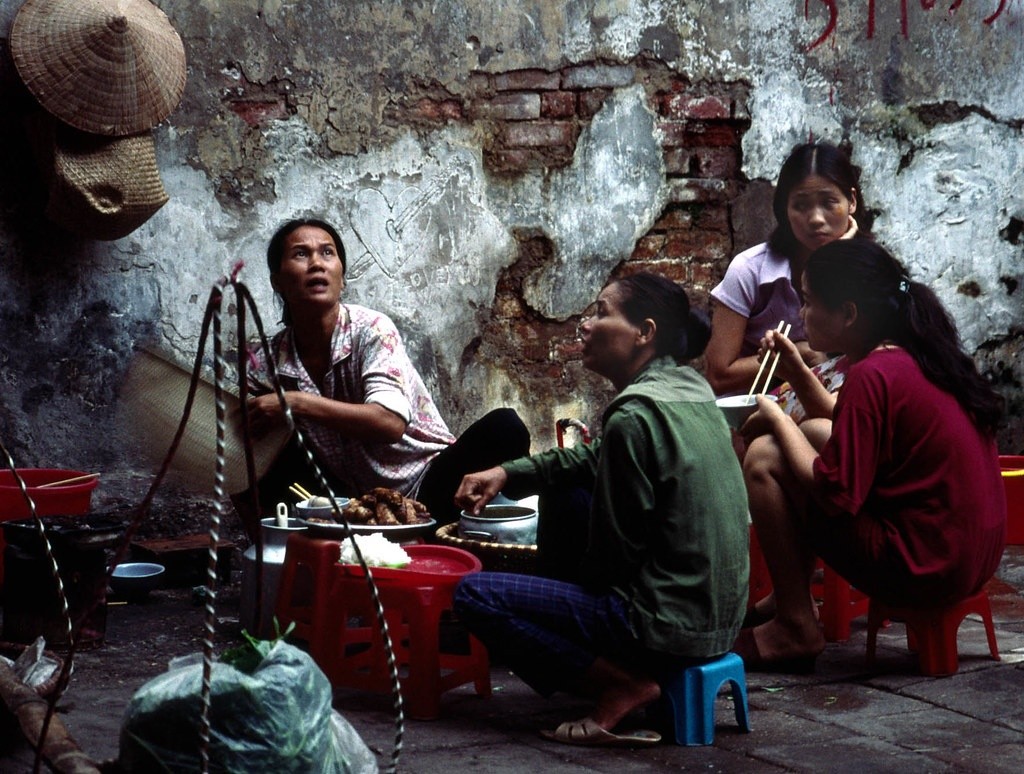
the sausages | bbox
[305,486,430,525]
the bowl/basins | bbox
[104,562,165,591]
[296,496,350,518]
[338,558,409,571]
[715,394,779,427]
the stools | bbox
[132,532,236,591]
[263,503,996,747]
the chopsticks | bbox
[288,480,313,499]
[743,321,792,405]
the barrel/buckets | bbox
[994,455,1024,548]
[0,468,99,518]
[240,517,308,635]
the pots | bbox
[461,503,539,544]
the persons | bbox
[456,271,752,750]
[706,144,880,396]
[742,238,1005,671]
[248,219,530,526]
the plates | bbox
[291,514,438,536]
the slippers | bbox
[543,718,663,745]
[736,627,815,675]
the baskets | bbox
[121,343,296,495]
[0,641,68,703]
[44,131,169,244]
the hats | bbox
[11,1,186,136]
